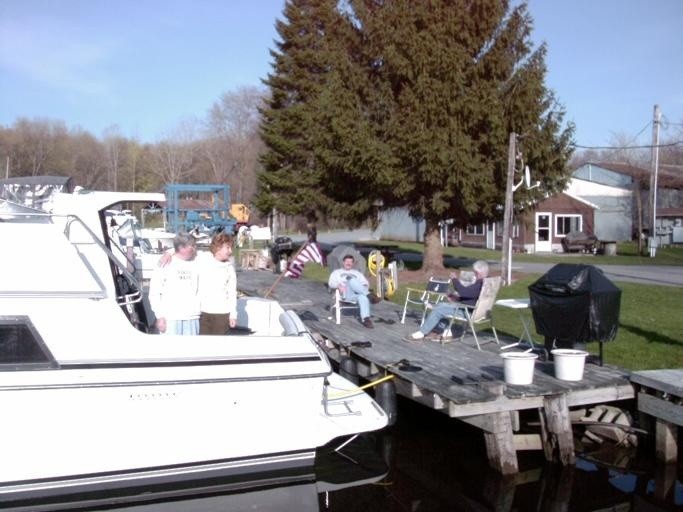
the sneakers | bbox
[403,335,453,342]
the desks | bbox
[495,298,541,359]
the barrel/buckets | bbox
[550,348,590,383]
[499,352,538,386]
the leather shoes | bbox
[367,294,381,304]
[363,317,372,328]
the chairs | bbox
[329,270,501,350]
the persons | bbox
[402,258,488,340]
[328,255,380,328]
[235,224,250,247]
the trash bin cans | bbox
[275,236,292,273]
[603,242,616,256]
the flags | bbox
[292,242,326,270]
[282,260,304,280]
